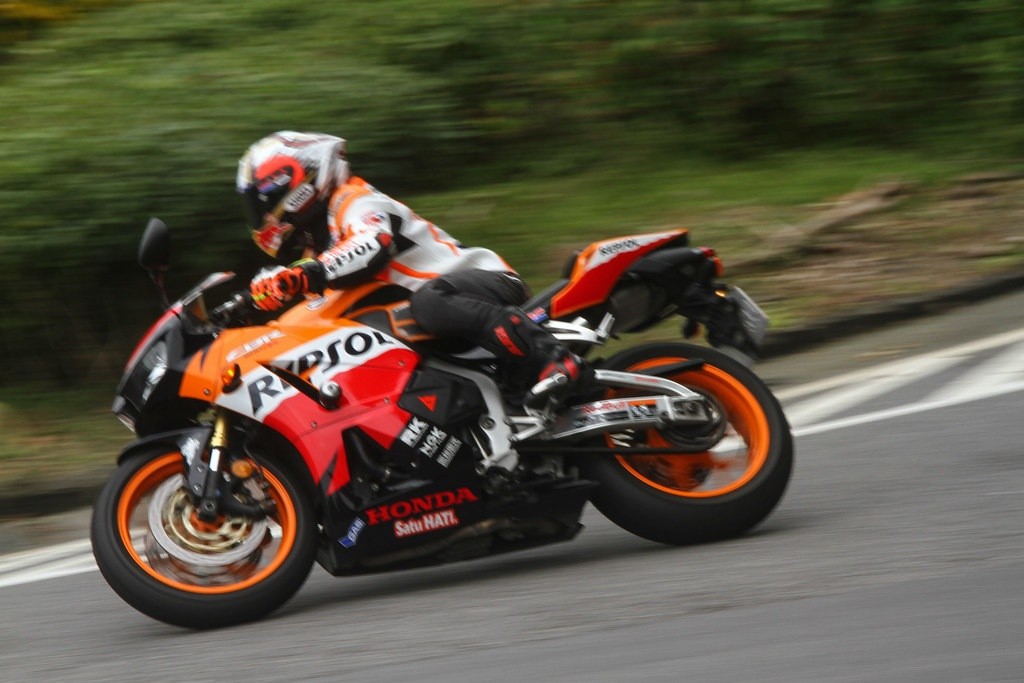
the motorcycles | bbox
[91,218,795,629]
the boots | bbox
[477,304,596,410]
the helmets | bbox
[237,130,351,258]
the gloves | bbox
[249,265,309,312]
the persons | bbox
[237,129,583,410]
